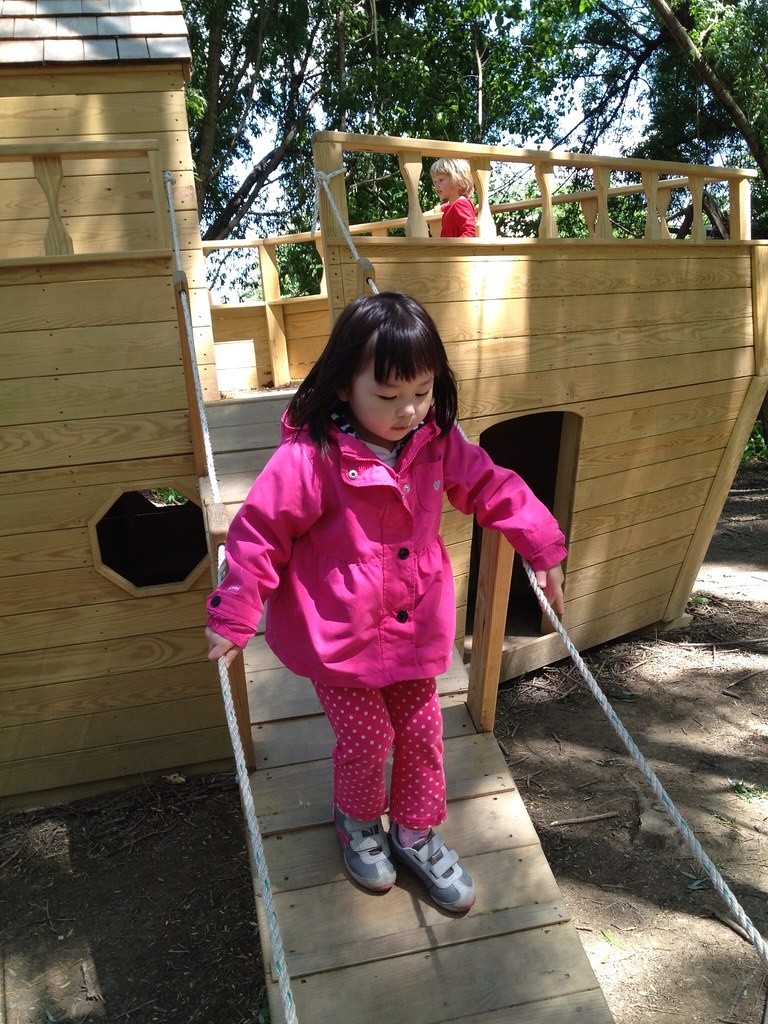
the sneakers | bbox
[332,802,397,892]
[388,821,476,912]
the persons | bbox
[429,157,477,239]
[202,289,567,911]
